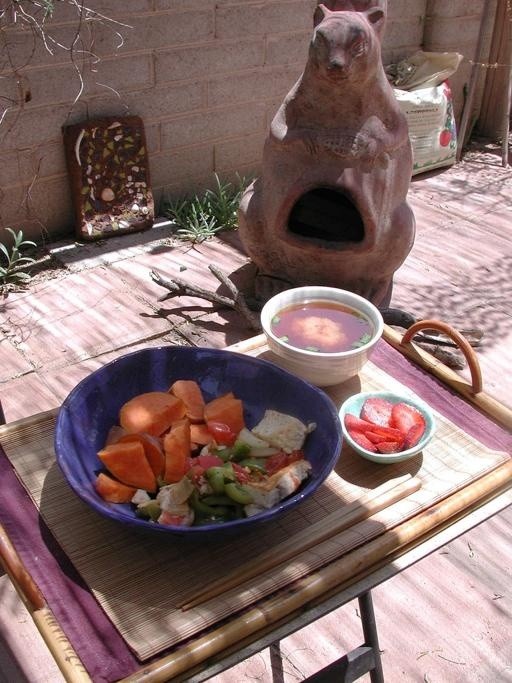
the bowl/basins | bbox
[258,284,383,386]
[54,345,342,542]
[340,390,434,464]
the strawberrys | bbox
[344,399,426,454]
